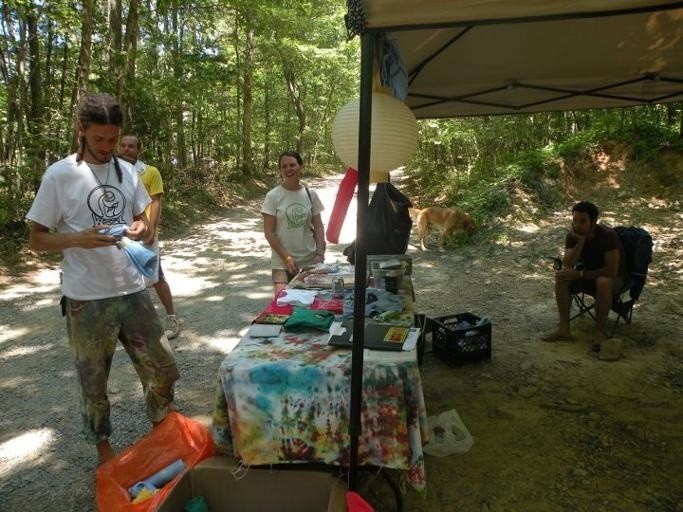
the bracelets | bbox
[579,270,583,279]
[284,257,292,265]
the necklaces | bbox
[85,155,110,198]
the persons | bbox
[25,93,180,467]
[118,131,177,337]
[537,201,628,352]
[260,152,325,296]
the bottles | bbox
[461,319,472,330]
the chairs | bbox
[570,225,653,335]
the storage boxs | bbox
[156,457,348,511]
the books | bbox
[328,317,421,351]
[306,274,370,288]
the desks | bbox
[215,262,425,511]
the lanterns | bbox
[331,83,418,184]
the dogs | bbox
[408,207,477,252]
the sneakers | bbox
[591,336,602,352]
[166,315,179,339]
[540,329,571,343]
